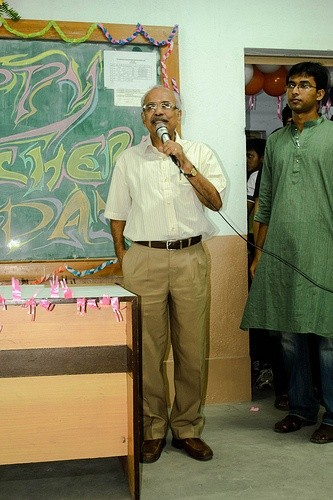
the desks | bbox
[0,285,142,500]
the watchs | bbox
[184,165,197,177]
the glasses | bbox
[286,82,316,90]
[141,102,178,110]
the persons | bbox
[246,103,292,410]
[104,86,227,464]
[240,61,333,443]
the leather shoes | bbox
[172,436,213,462]
[140,439,166,464]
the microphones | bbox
[155,123,177,163]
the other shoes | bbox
[253,359,274,391]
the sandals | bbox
[311,424,333,443]
[275,415,317,432]
[274,394,290,411]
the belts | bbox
[133,236,202,251]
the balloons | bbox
[244,64,288,97]
